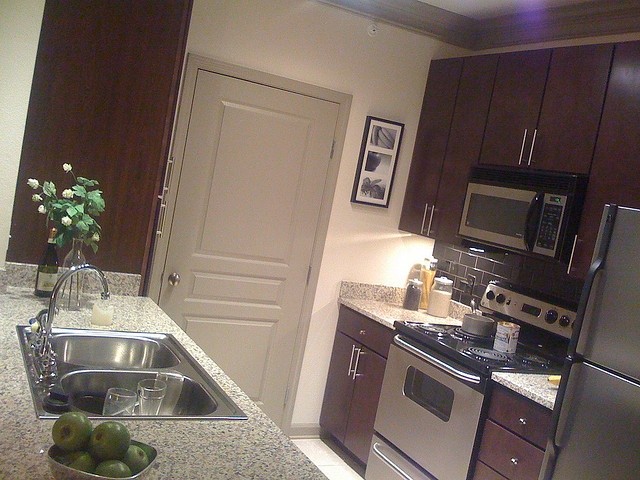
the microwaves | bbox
[456,166,584,262]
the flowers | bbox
[27,163,106,254]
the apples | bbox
[54,450,96,472]
[89,421,130,459]
[52,411,92,452]
[95,460,131,477]
[123,445,150,473]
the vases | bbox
[55,238,89,309]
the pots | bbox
[463,298,494,334]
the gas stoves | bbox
[414,321,551,367]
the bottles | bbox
[402,279,423,310]
[35,230,60,297]
[419,256,438,309]
[427,277,453,318]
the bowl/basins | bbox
[47,438,159,479]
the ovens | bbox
[365,330,492,480]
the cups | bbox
[155,375,184,414]
[103,387,136,417]
[138,380,166,417]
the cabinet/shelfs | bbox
[478,41,615,175]
[319,305,395,466]
[471,384,555,480]
[399,53,498,245]
[565,40,640,282]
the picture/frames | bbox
[352,117,405,209]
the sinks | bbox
[51,333,180,370]
[59,372,217,417]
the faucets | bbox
[44,263,111,330]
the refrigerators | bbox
[537,201,640,479]
